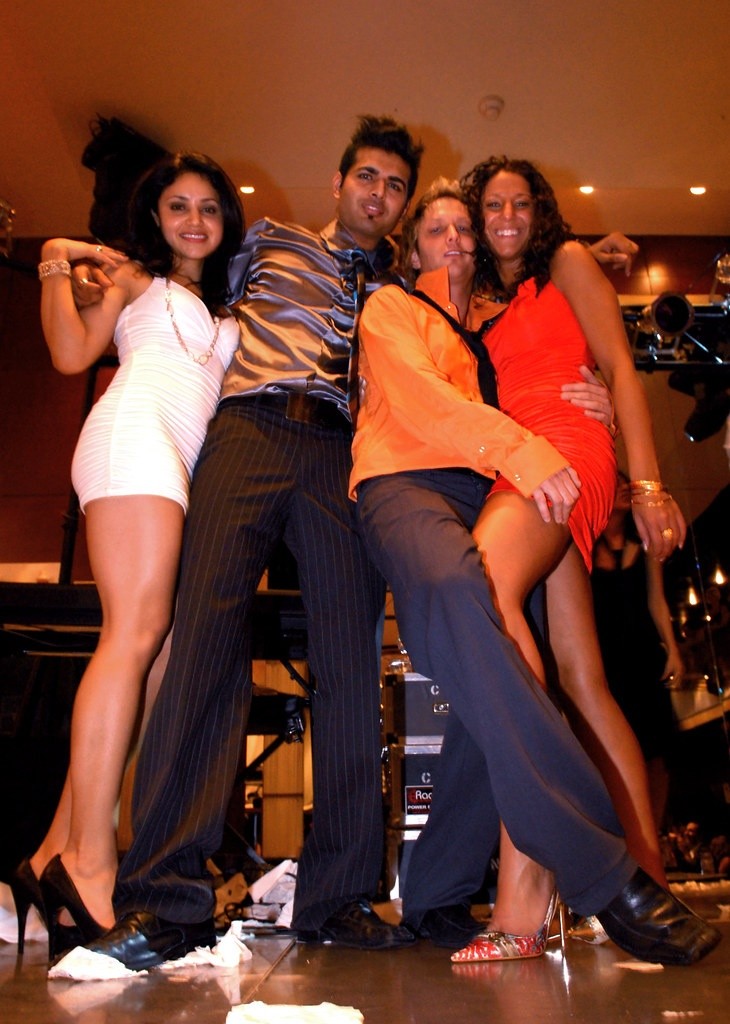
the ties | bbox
[418,293,498,409]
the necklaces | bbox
[165,277,220,366]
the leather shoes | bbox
[297,904,416,948]
[400,906,486,946]
[55,914,216,972]
[595,866,722,963]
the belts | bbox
[248,391,348,432]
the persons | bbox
[10,152,246,962]
[450,155,687,963]
[48,115,639,973]
[349,176,723,966]
[592,468,684,829]
[658,822,730,875]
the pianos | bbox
[1,579,315,662]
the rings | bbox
[662,528,673,540]
[77,278,88,288]
[96,246,102,252]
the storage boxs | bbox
[399,830,423,897]
[404,673,449,745]
[401,746,443,826]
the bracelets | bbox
[38,259,72,280]
[630,480,673,507]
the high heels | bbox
[451,887,609,962]
[9,853,114,961]
